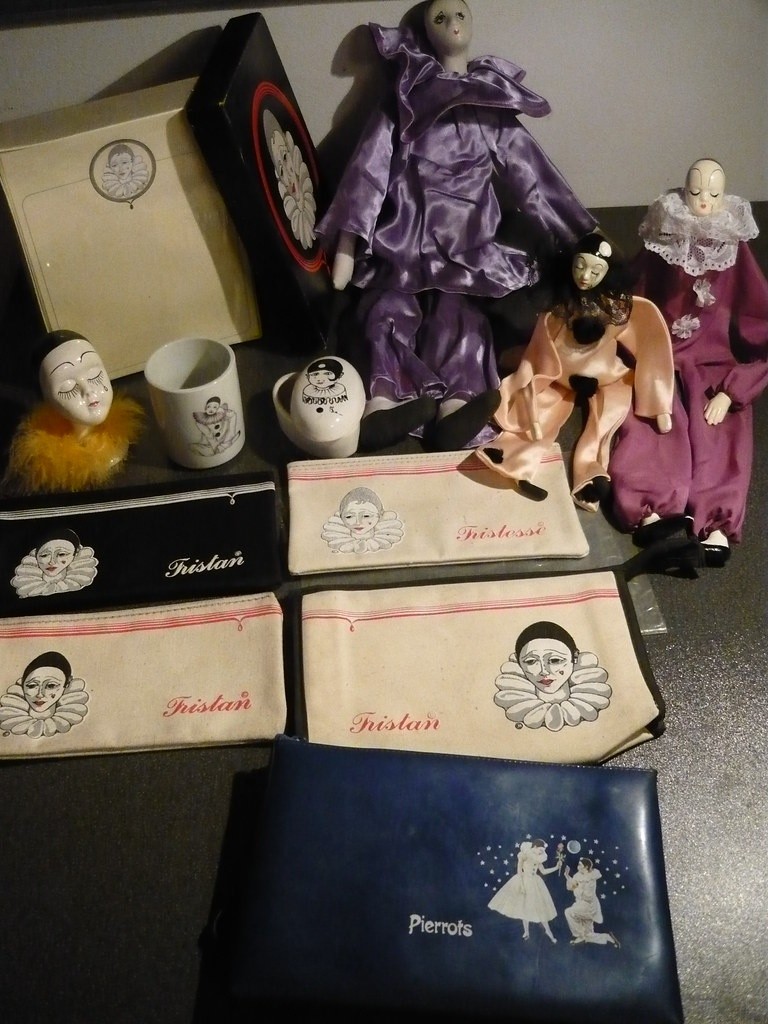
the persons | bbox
[610,157,768,565]
[9,329,143,493]
[313,0,622,450]
[476,232,675,513]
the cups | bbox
[144,338,245,468]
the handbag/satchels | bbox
[225,729,684,1024]
